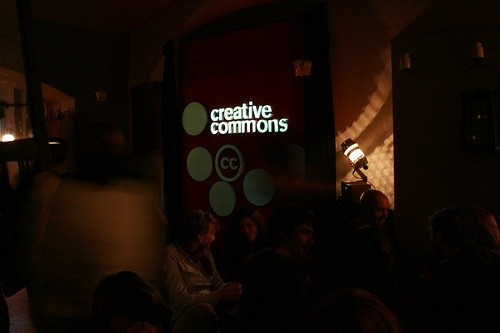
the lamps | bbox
[341,138,368,182]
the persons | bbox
[440,206,500,332]
[225,209,272,275]
[311,290,400,333]
[345,190,418,319]
[424,207,466,276]
[245,206,320,333]
[92,271,171,333]
[163,208,244,333]
